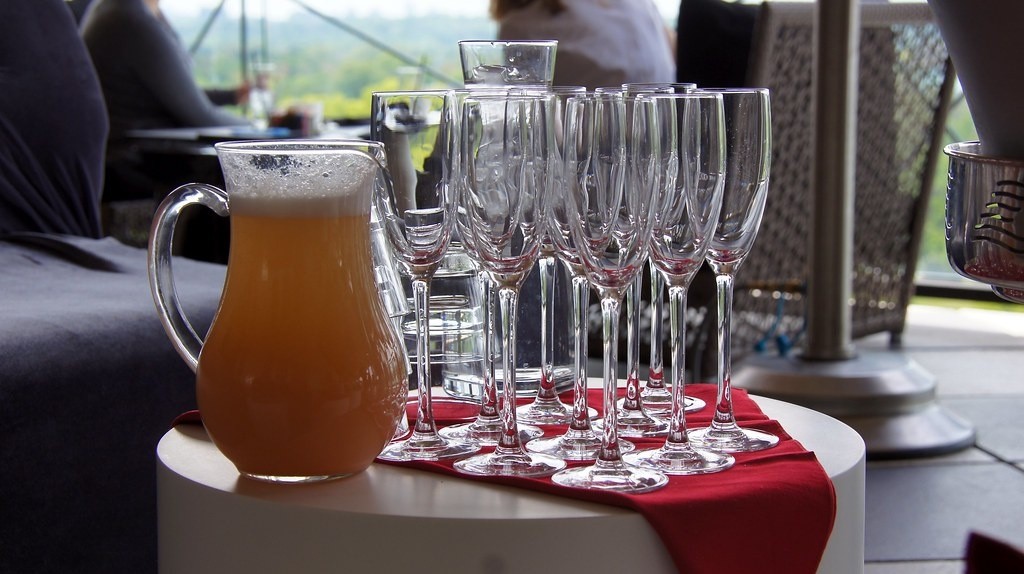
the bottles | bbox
[442,42,587,399]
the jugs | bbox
[147,140,406,482]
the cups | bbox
[249,63,274,111]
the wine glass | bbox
[369,82,778,492]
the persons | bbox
[493,0,677,91]
[77,0,252,261]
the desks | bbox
[155,376,867,573]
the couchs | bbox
[568,1,897,315]
[0,0,252,574]
